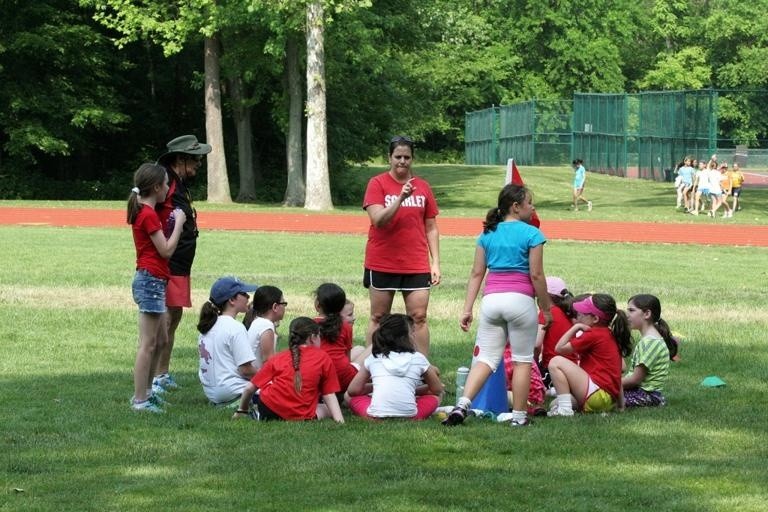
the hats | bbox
[211,277,258,304]
[573,296,613,320]
[158,135,212,165]
[545,276,568,297]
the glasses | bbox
[277,302,287,306]
[390,136,413,145]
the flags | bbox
[505,157,541,229]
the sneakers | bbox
[160,375,177,387]
[442,397,471,424]
[672,205,743,219]
[152,377,167,393]
[147,391,171,406]
[131,398,166,413]
[547,402,575,416]
[587,201,592,211]
[510,410,529,426]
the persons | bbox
[444,182,556,427]
[194,277,261,407]
[672,153,747,219]
[569,158,593,214]
[343,315,443,424]
[151,132,213,400]
[241,283,359,421]
[365,134,441,364]
[126,161,185,414]
[503,276,680,419]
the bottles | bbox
[455,366,469,401]
